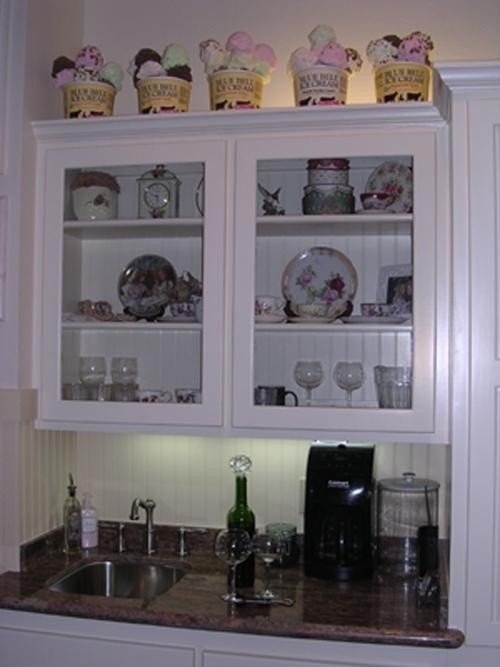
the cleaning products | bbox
[63,473,81,554]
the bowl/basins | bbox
[65,381,139,400]
[359,192,393,210]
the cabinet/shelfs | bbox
[0,613,452,665]
[28,103,454,446]
[448,80,500,650]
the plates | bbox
[254,315,287,323]
[118,254,177,317]
[359,209,395,215]
[289,316,336,323]
[156,315,197,322]
[365,160,415,215]
[340,315,409,325]
[280,245,358,319]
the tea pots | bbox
[111,357,138,402]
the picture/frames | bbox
[374,259,411,319]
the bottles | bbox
[226,474,256,589]
[376,469,442,579]
[69,172,120,221]
[62,483,81,553]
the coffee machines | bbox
[302,440,375,583]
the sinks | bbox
[44,553,192,600]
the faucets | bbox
[130,498,158,555]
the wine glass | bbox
[334,360,365,407]
[255,526,286,598]
[294,360,323,406]
[217,530,253,601]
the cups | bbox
[375,364,412,407]
[135,388,171,403]
[175,386,198,402]
[267,522,295,557]
[296,303,329,317]
[78,355,107,401]
[171,301,193,316]
[258,385,298,405]
[360,302,401,316]
[256,295,285,315]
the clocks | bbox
[137,164,182,219]
